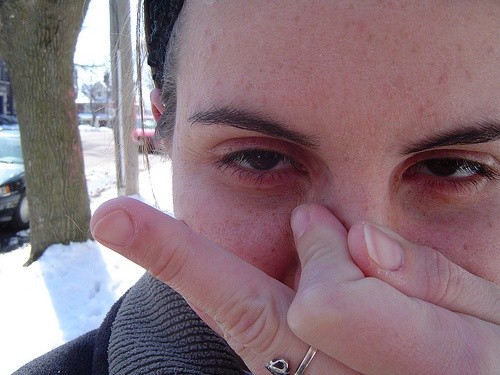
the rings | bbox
[266,345,318,375]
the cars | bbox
[133,116,163,153]
[0,163,31,232]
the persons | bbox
[12,0,500,375]
